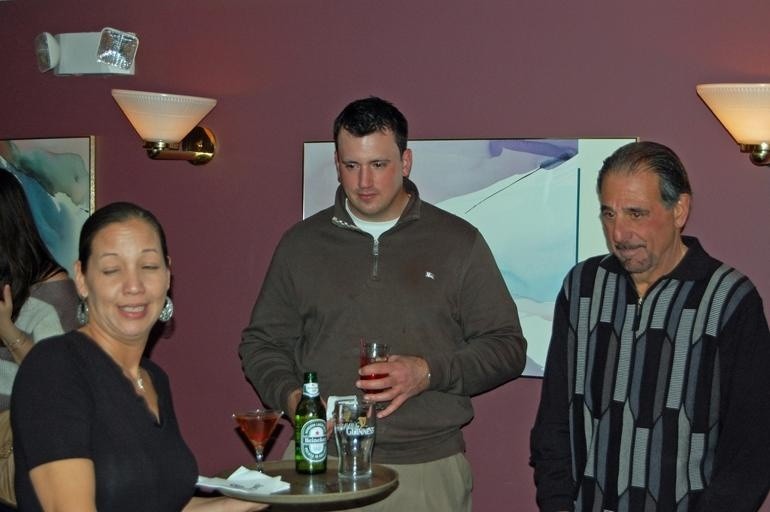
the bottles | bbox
[293,372,327,476]
[290,474,328,499]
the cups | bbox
[358,338,391,395]
[337,476,373,493]
[334,400,377,481]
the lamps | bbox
[692,80,770,169]
[32,27,141,77]
[110,89,219,166]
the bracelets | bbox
[4,330,29,354]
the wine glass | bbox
[230,409,284,478]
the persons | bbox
[527,143,769,512]
[8,202,269,511]
[0,167,85,507]
[239,96,527,512]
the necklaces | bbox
[122,365,147,392]
